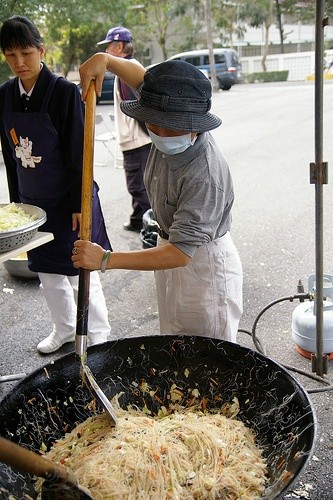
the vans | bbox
[163,48,244,91]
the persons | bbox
[97,28,152,232]
[0,16,113,353]
[72,53,243,344]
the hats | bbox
[97,27,131,45]
[119,61,222,132]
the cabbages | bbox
[40,369,254,500]
[0,202,40,232]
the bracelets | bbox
[101,250,111,273]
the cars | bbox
[77,71,114,106]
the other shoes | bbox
[36,329,76,353]
[123,221,142,233]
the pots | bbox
[0,334,316,500]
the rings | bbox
[72,247,77,254]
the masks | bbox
[146,127,198,155]
